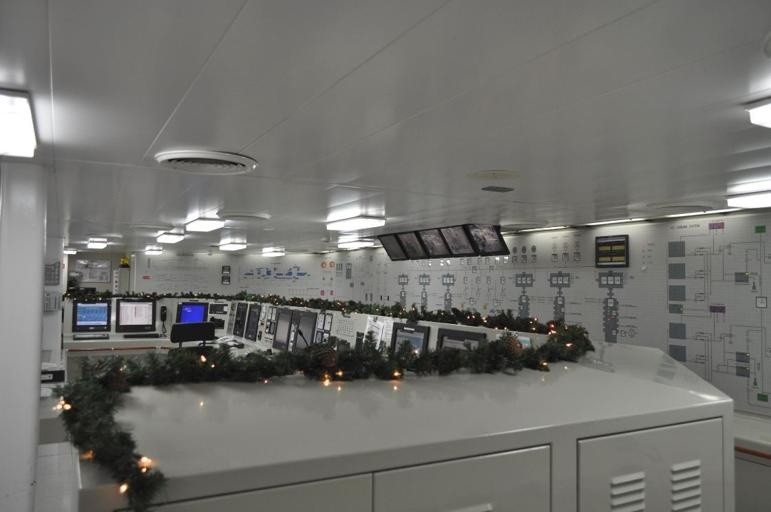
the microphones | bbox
[296,328,310,347]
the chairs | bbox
[171,322,217,346]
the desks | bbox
[64,330,273,384]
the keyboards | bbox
[123,333,160,338]
[73,333,110,339]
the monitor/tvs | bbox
[245,303,262,342]
[177,300,209,324]
[390,322,429,357]
[396,231,430,261]
[595,235,629,268]
[433,327,485,354]
[293,312,316,351]
[270,307,293,350]
[376,232,410,262]
[72,300,111,332]
[468,223,511,257]
[415,229,451,260]
[115,300,154,331]
[233,303,248,337]
[438,227,476,257]
